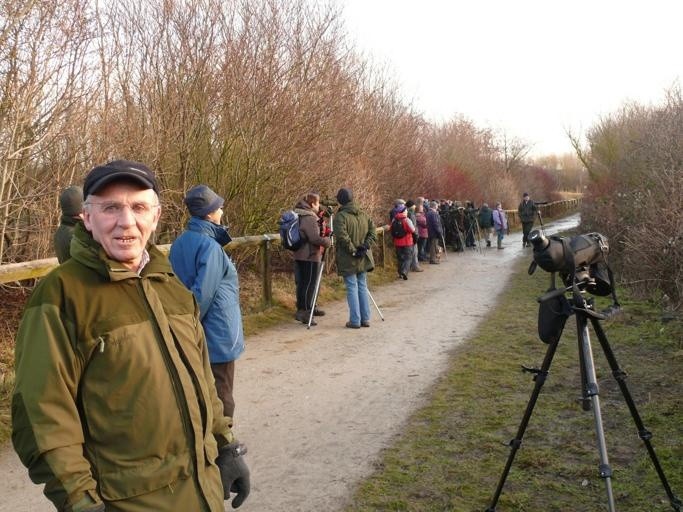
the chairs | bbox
[296,307,325,326]
[346,322,370,328]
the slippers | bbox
[276,210,301,252]
[391,220,406,239]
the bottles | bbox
[60,185,84,216]
[184,185,225,217]
[337,189,353,205]
[397,203,406,213]
[84,160,160,201]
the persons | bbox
[53,185,85,265]
[332,188,377,328]
[291,193,331,327]
[479,203,495,247]
[10,159,251,512]
[168,184,247,455]
[518,192,540,248]
[389,196,477,280]
[492,202,508,250]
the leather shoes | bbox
[356,244,368,257]
[215,440,250,508]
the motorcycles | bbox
[531,204,545,234]
[487,270,683,512]
[307,206,385,330]
[437,212,482,262]
